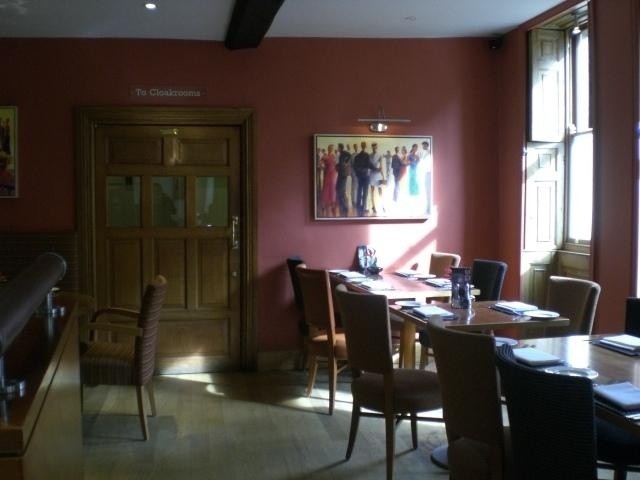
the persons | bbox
[317,142,431,217]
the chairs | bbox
[430,251,461,275]
[78,274,167,440]
[625,298,640,339]
[334,283,443,480]
[425,320,508,480]
[496,344,598,480]
[295,263,349,416]
[420,259,508,370]
[286,258,343,371]
[544,276,601,334]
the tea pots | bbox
[449,267,473,310]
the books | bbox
[513,358,570,370]
[590,340,639,358]
[489,304,515,315]
[406,310,459,321]
[594,397,640,421]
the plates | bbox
[522,310,560,319]
[345,277,372,283]
[546,365,599,381]
[414,274,438,279]
[395,300,422,309]
[494,334,518,350]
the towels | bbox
[362,281,390,289]
[413,306,454,317]
[425,279,452,287]
[593,382,640,411]
[340,272,366,278]
[495,302,538,312]
[512,348,561,366]
[396,270,420,276]
[600,334,640,351]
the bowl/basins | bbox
[367,266,383,273]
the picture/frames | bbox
[313,133,432,221]
[0,106,19,199]
